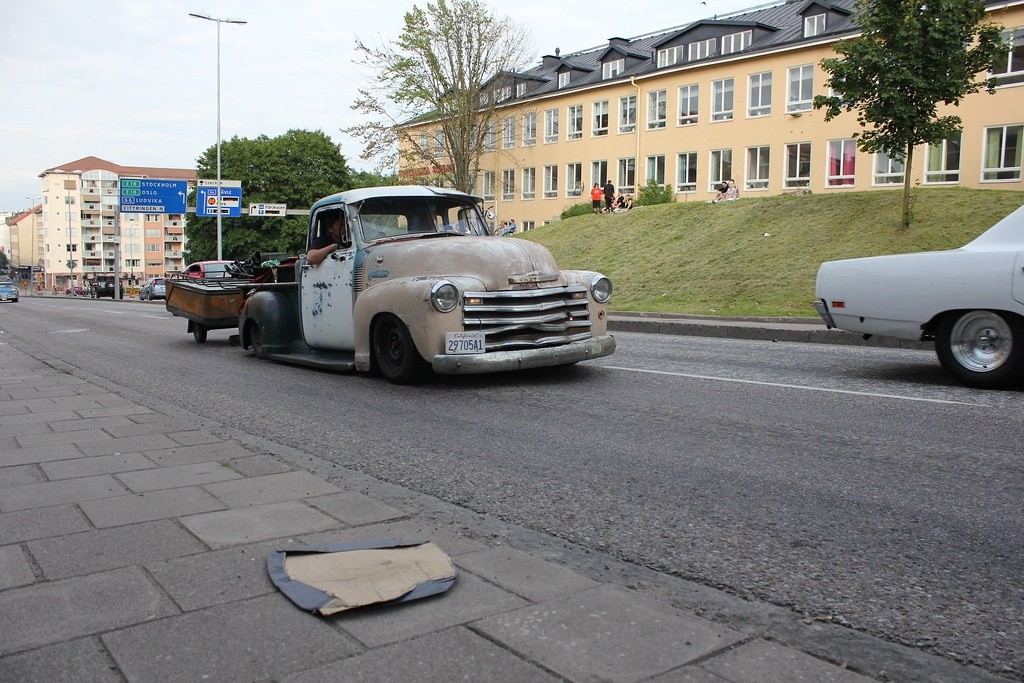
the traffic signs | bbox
[250,202,287,218]
[119,177,188,215]
[196,179,243,218]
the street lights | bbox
[55,169,83,294]
[188,12,247,262]
[25,196,42,295]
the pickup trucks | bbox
[66,287,85,296]
[166,181,616,383]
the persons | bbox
[306,212,344,263]
[713,179,739,204]
[617,191,633,212]
[591,183,602,214]
[604,180,614,212]
[495,218,517,237]
[714,181,729,199]
[53,284,88,297]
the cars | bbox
[0,281,20,302]
[172,261,244,279]
[816,203,1024,388]
[139,276,166,301]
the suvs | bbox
[90,276,124,300]
